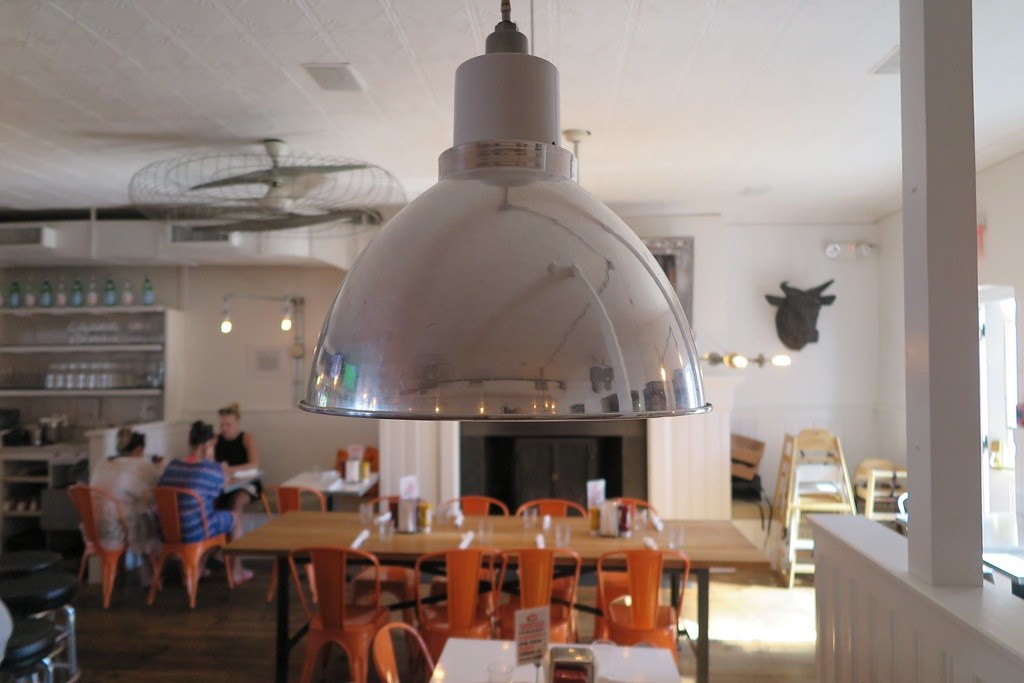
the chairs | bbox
[422,496,511,629]
[287,546,393,683]
[593,549,690,669]
[349,496,430,626]
[260,483,330,605]
[335,443,378,508]
[142,487,235,609]
[372,622,436,683]
[66,482,163,607]
[491,545,582,645]
[499,499,588,646]
[594,497,666,643]
[413,545,503,683]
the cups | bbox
[358,502,373,525]
[436,503,449,524]
[379,519,393,541]
[479,519,494,544]
[523,507,537,529]
[555,522,571,547]
[486,661,514,683]
[668,523,685,550]
[592,639,617,678]
[633,508,647,533]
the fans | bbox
[128,140,408,241]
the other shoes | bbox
[235,569,253,586]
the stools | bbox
[0,550,82,683]
[772,426,909,590]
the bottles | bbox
[618,505,631,538]
[588,506,599,536]
[8,271,154,306]
[64,319,165,344]
[417,501,432,532]
[42,360,164,389]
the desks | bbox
[227,467,772,683]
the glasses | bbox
[218,408,238,416]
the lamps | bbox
[220,291,233,333]
[281,294,302,331]
[297,0,715,424]
[706,351,749,370]
[759,354,792,368]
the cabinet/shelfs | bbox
[0,305,187,586]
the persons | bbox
[214,403,264,512]
[89,428,157,589]
[157,420,253,586]
